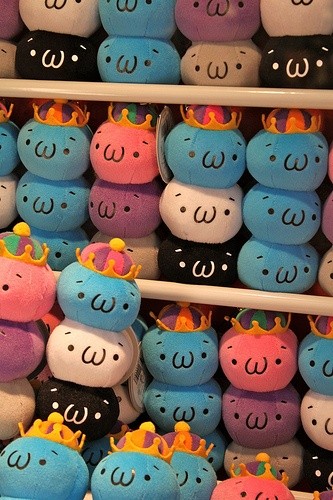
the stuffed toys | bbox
[0,99,333,301]
[0,0,333,91]
[0,221,333,500]
